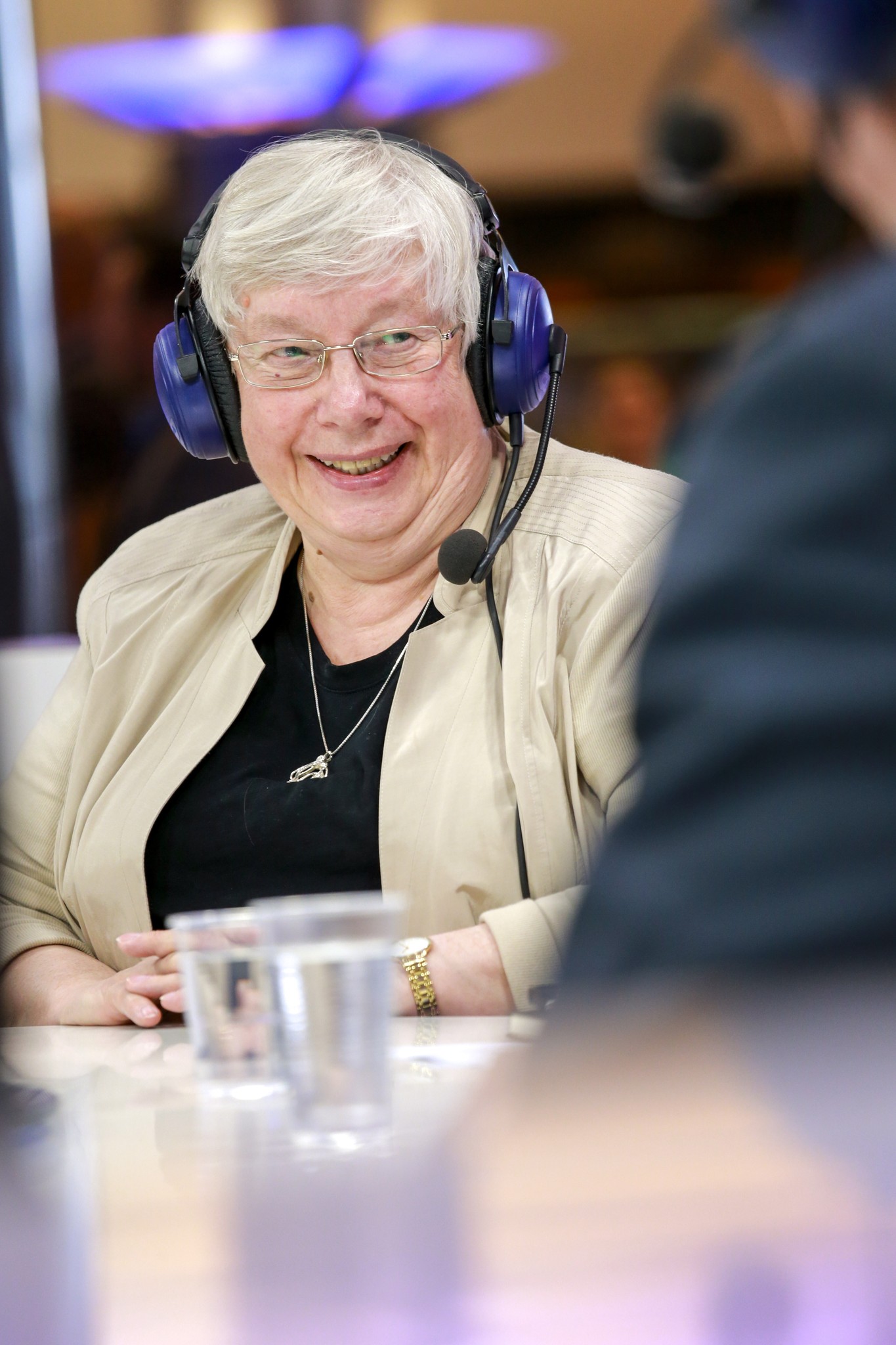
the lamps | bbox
[41,12,550,182]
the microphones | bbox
[436,409,528,585]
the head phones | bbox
[151,123,565,467]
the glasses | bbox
[224,325,463,390]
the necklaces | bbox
[286,557,436,786]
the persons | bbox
[532,0,892,1086]
[0,125,707,1015]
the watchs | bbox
[392,933,442,1016]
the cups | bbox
[171,901,282,1088]
[253,883,400,1158]
